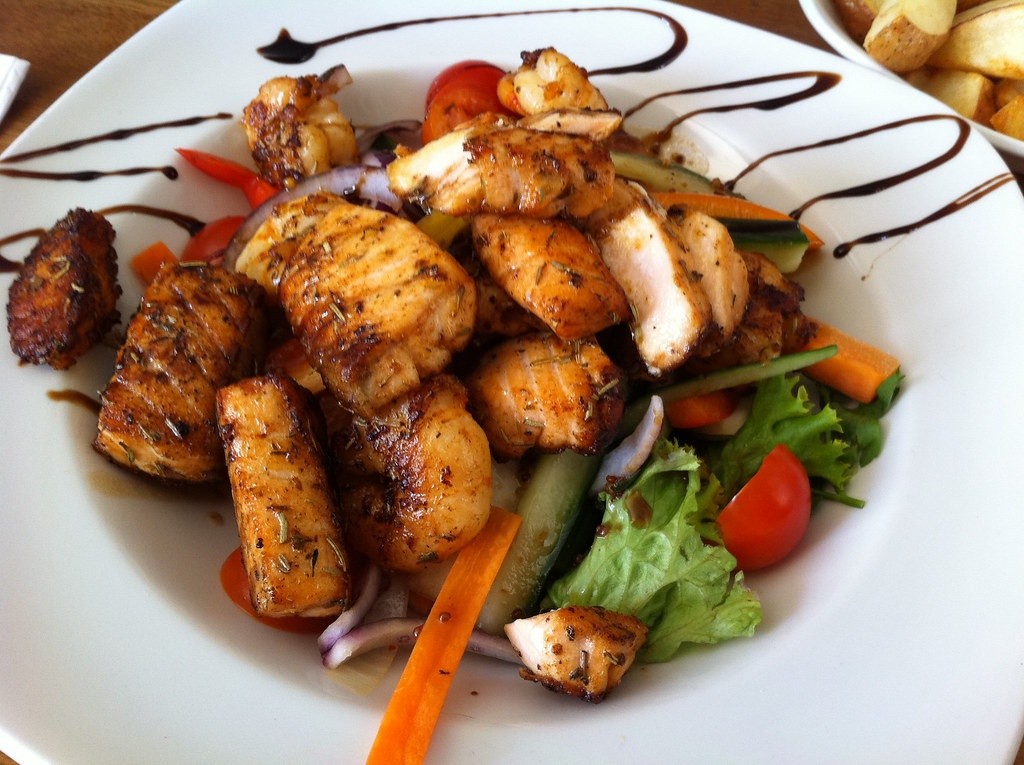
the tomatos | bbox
[424,62,517,143]
[714,442,812,569]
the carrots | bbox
[366,506,521,765]
[648,190,822,252]
[796,315,896,403]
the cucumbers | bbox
[476,417,599,633]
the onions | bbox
[320,562,527,673]
[224,117,428,271]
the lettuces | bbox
[545,373,865,658]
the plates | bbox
[0,1,1024,764]
